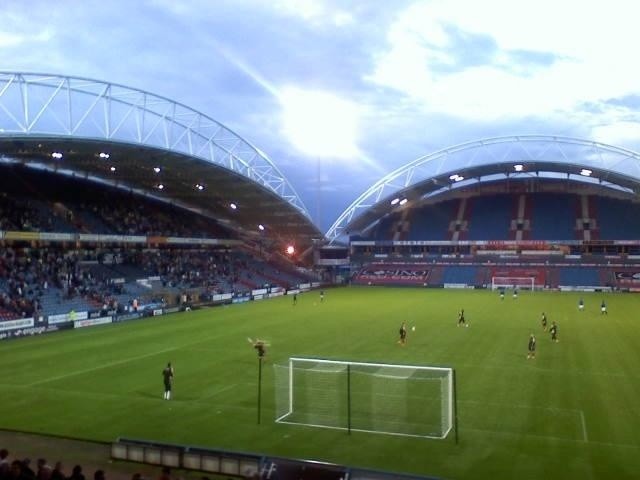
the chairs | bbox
[0,312,19,322]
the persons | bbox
[456,308,469,329]
[512,289,517,298]
[500,287,504,301]
[548,319,560,343]
[247,338,270,363]
[0,192,299,322]
[396,319,409,346]
[578,297,584,313]
[161,361,175,402]
[598,300,608,315]
[541,311,547,332]
[0,448,108,480]
[526,334,536,359]
[318,290,326,303]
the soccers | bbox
[411,327,415,331]
